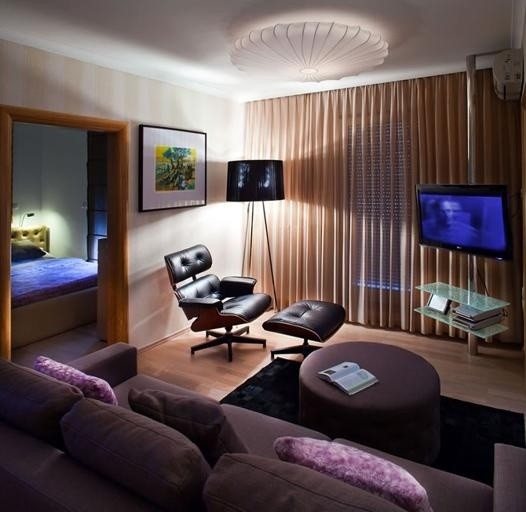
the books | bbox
[317,361,379,396]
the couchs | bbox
[1,340,526,512]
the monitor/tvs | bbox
[415,183,515,262]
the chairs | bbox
[165,244,270,358]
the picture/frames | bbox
[139,125,207,214]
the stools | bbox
[300,341,442,465]
[264,300,345,359]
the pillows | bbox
[11,238,47,260]
[33,354,116,404]
[128,388,247,463]
[273,436,433,510]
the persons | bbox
[422,198,481,247]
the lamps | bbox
[226,160,285,314]
[229,21,389,83]
[21,212,34,227]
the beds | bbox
[11,226,100,351]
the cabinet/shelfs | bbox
[413,282,510,358]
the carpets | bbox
[221,356,526,488]
